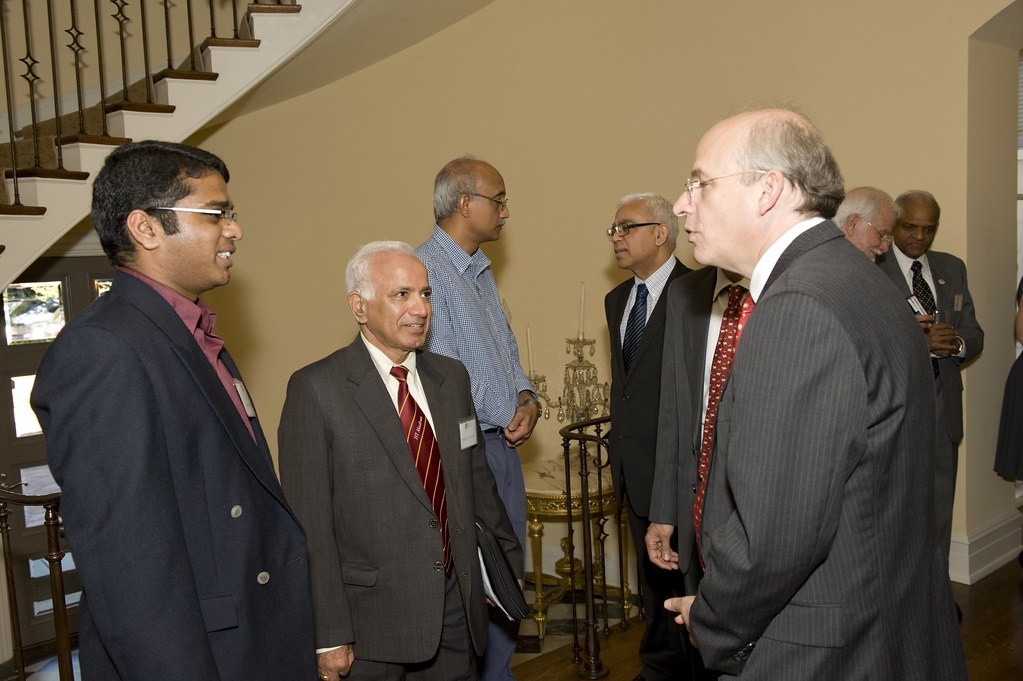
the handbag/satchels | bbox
[473,515,531,620]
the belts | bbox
[480,425,506,436]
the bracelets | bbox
[954,338,962,355]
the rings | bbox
[320,675,329,680]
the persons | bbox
[604,193,697,681]
[29,139,318,681]
[876,190,985,622]
[663,107,970,680]
[414,158,543,681]
[834,187,903,261]
[994,277,1023,483]
[646,262,756,599]
[277,241,524,681]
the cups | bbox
[932,308,953,356]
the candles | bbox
[579,279,586,340]
[526,323,535,378]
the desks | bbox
[516,458,632,642]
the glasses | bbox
[608,222,661,242]
[859,215,895,246]
[145,206,239,226]
[460,193,509,212]
[685,170,768,205]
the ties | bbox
[694,292,757,572]
[698,285,748,478]
[388,364,453,580]
[910,261,940,380]
[622,283,649,376]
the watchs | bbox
[521,398,542,417]
